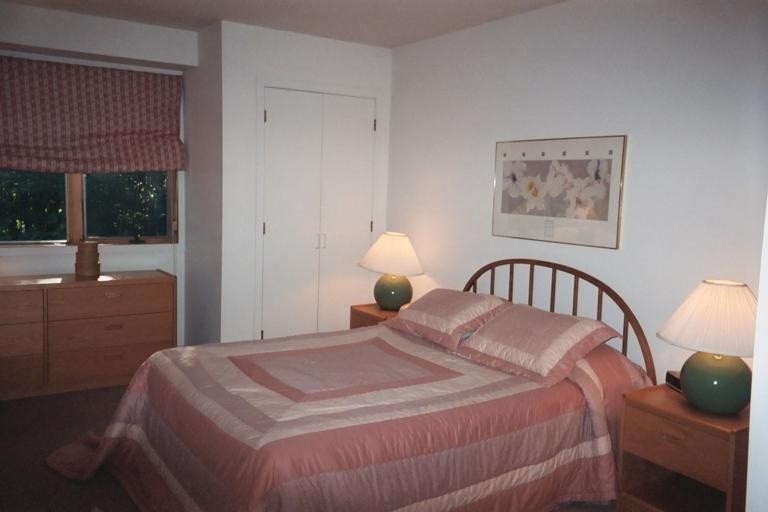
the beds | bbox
[46,257,656,509]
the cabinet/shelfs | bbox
[2,275,46,401]
[260,82,378,344]
[42,268,177,395]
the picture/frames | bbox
[489,135,627,252]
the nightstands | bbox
[612,378,750,512]
[347,302,398,328]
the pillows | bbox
[385,286,512,351]
[456,301,623,384]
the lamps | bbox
[357,232,426,309]
[652,278,758,419]
